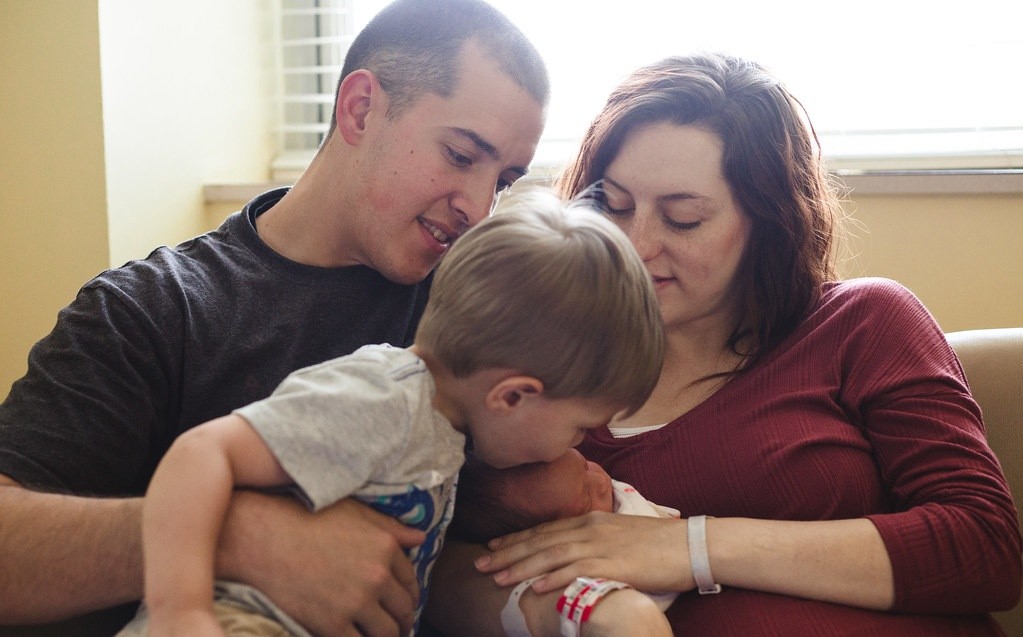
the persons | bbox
[0,0,1023,637]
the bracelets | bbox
[500,573,548,637]
[556,576,634,637]
[688,516,722,595]
